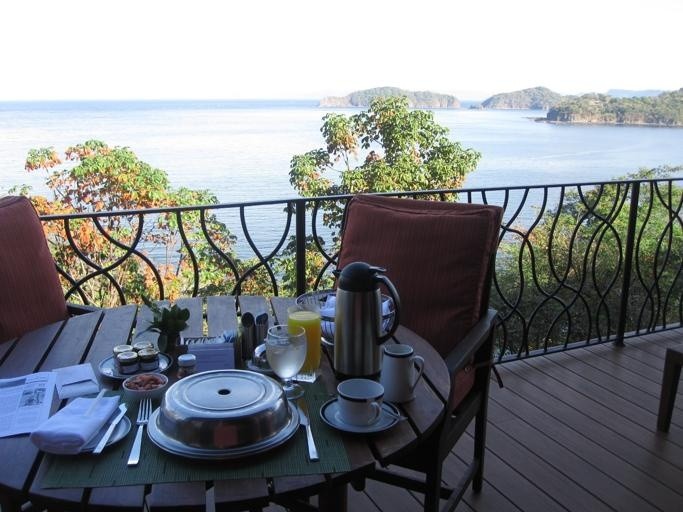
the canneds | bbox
[112,341,159,373]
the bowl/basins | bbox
[121,372,168,401]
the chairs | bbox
[0,193,100,349]
[276,193,504,511]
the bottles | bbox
[138,349,159,371]
[115,352,138,375]
[177,353,197,379]
[133,341,154,352]
[112,344,133,356]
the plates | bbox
[319,396,399,436]
[96,350,174,380]
[82,409,131,455]
[245,359,277,374]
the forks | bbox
[125,396,154,467]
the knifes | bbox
[295,396,319,462]
[92,400,131,456]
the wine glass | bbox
[264,325,307,401]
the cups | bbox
[376,342,424,404]
[283,304,323,385]
[334,378,384,426]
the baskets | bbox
[295,289,395,347]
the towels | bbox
[28,395,123,456]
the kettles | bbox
[328,261,401,380]
